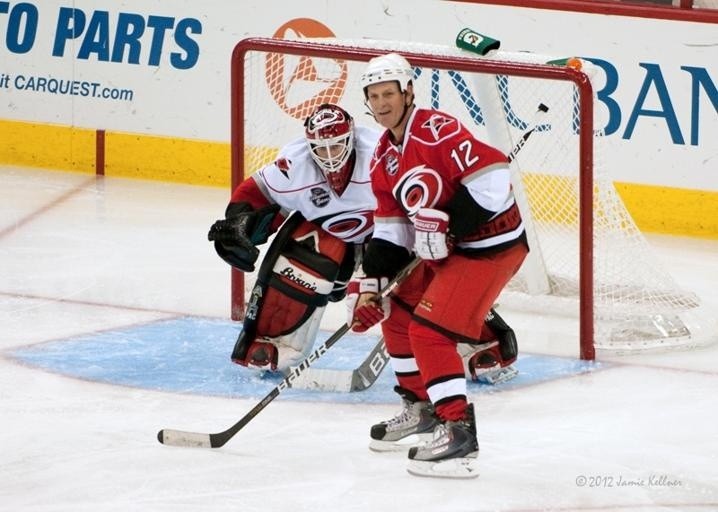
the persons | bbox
[469,34,480,46]
[349,51,530,465]
[208,102,520,385]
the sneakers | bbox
[406,403,482,460]
[367,384,441,441]
[473,349,503,375]
[247,340,274,364]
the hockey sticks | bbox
[157,259,425,448]
[284,104,550,393]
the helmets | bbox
[360,52,415,105]
[303,103,356,173]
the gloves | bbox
[346,275,386,333]
[414,206,455,268]
[208,212,258,273]
